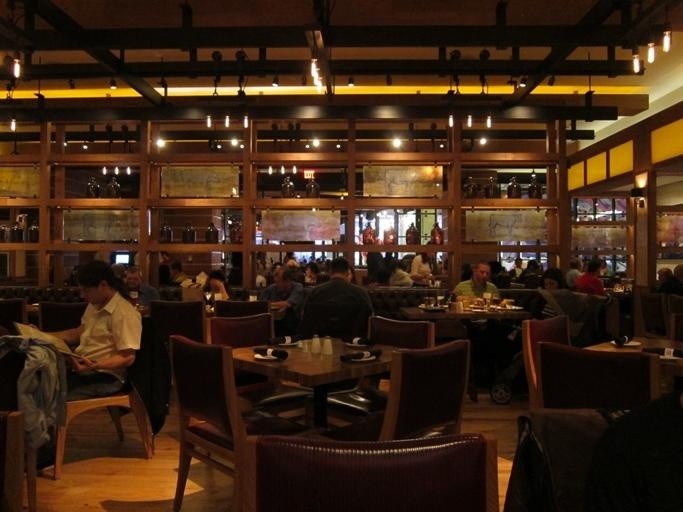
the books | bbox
[14,321,97,363]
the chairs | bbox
[537,341,661,411]
[215,300,271,317]
[0,299,29,334]
[609,406,683,512]
[206,314,315,428]
[306,315,435,428]
[171,334,324,512]
[242,435,499,512]
[522,315,606,474]
[144,300,208,413]
[502,410,609,512]
[311,340,469,443]
[38,301,88,331]
[0,411,37,512]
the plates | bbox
[610,340,642,346]
[351,356,376,361]
[255,353,278,360]
[278,342,299,346]
[346,342,367,346]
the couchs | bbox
[0,286,250,304]
[308,287,571,316]
[570,292,609,318]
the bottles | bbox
[87,177,99,198]
[107,178,120,197]
[323,336,333,355]
[364,223,376,244]
[207,223,218,243]
[384,226,396,244]
[485,177,498,198]
[305,179,320,198]
[184,223,195,243]
[431,223,443,244]
[507,177,521,198]
[282,177,295,198]
[311,335,321,354]
[462,177,477,198]
[407,223,419,244]
[28,220,39,243]
[161,226,173,242]
[528,178,542,199]
[10,222,22,243]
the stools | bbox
[54,386,154,479]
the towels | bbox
[342,336,373,345]
[268,335,302,348]
[642,347,683,358]
[615,334,633,348]
[252,348,288,360]
[340,349,382,362]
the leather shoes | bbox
[37,455,55,472]
[468,387,478,403]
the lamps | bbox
[111,76,117,89]
[648,18,655,63]
[82,138,88,150]
[348,75,355,87]
[439,137,444,149]
[519,75,527,89]
[662,5,672,53]
[10,112,16,129]
[13,46,21,78]
[336,137,340,149]
[272,75,279,86]
[631,189,644,208]
[632,36,640,73]
[548,75,555,86]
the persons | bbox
[452,261,515,402]
[512,257,540,280]
[411,241,437,285]
[512,267,577,390]
[305,258,373,341]
[306,262,327,285]
[125,266,158,315]
[369,258,413,285]
[579,258,606,294]
[21,261,140,472]
[657,264,683,294]
[565,259,580,289]
[257,257,304,328]
[202,271,233,301]
